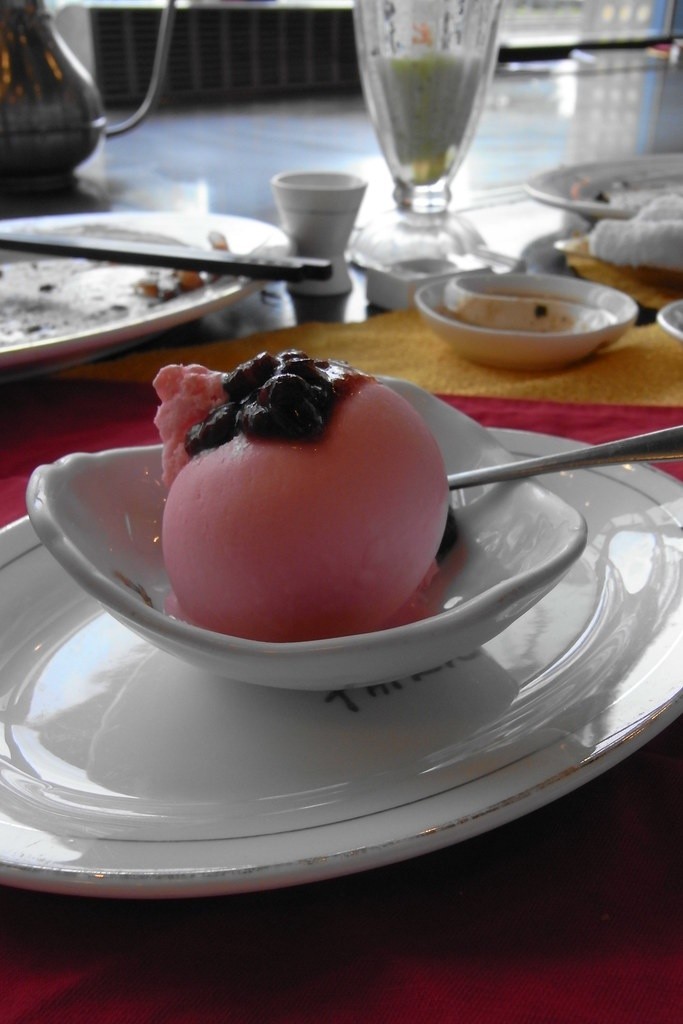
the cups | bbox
[272,172,369,296]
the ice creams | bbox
[153,344,459,646]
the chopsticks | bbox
[0,232,333,285]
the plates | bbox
[521,152,683,228]
[0,209,296,368]
[413,274,639,371]
[0,429,683,899]
[24,375,590,688]
[657,300,683,342]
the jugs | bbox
[0,0,179,187]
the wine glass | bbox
[352,0,509,275]
